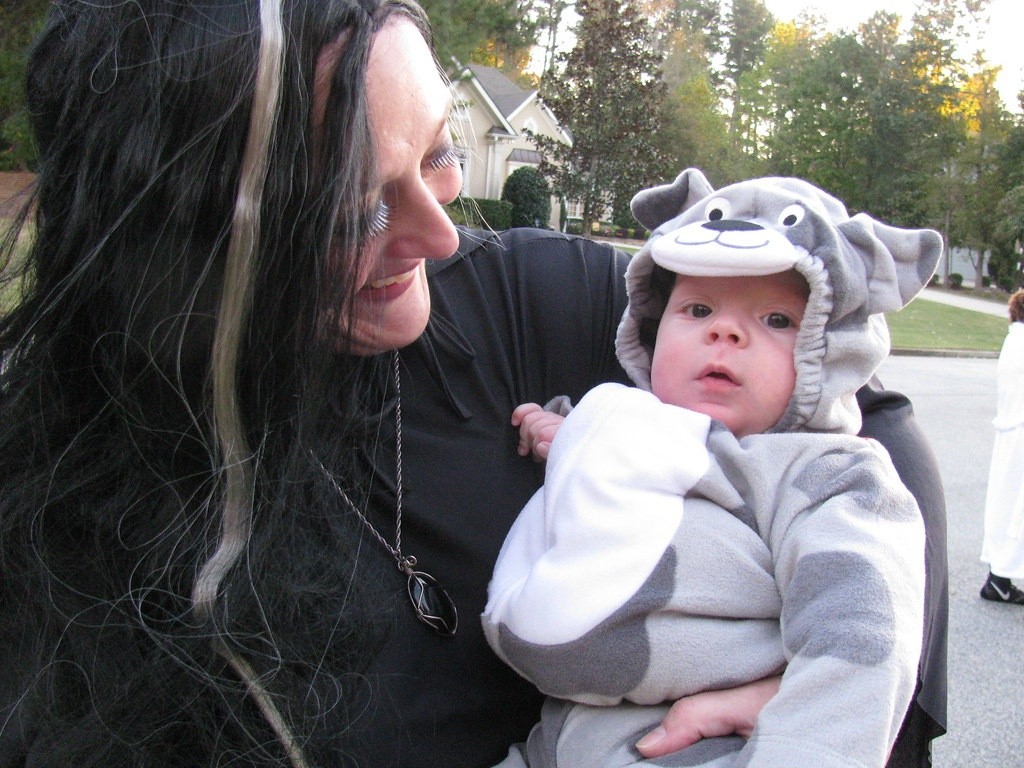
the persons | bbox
[981,289,1024,605]
[0,0,949,768]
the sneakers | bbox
[980,574,1024,605]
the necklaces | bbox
[305,351,459,635]
